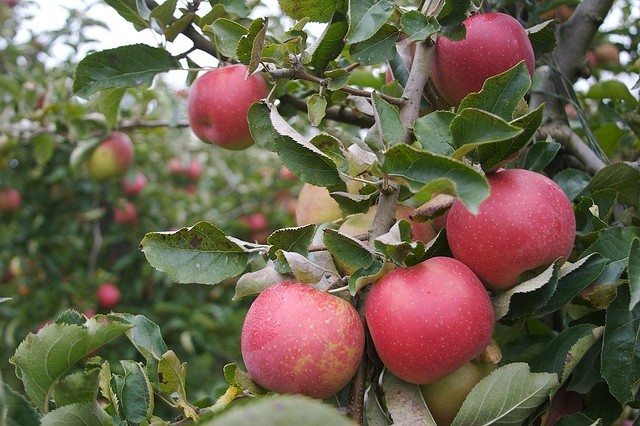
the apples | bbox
[429,190,453,233]
[240,280,366,400]
[419,337,502,426]
[550,5,620,120]
[165,153,298,261]
[447,169,574,294]
[427,14,537,105]
[1,74,157,349]
[299,164,382,249]
[334,203,436,280]
[367,257,494,385]
[189,63,270,152]
